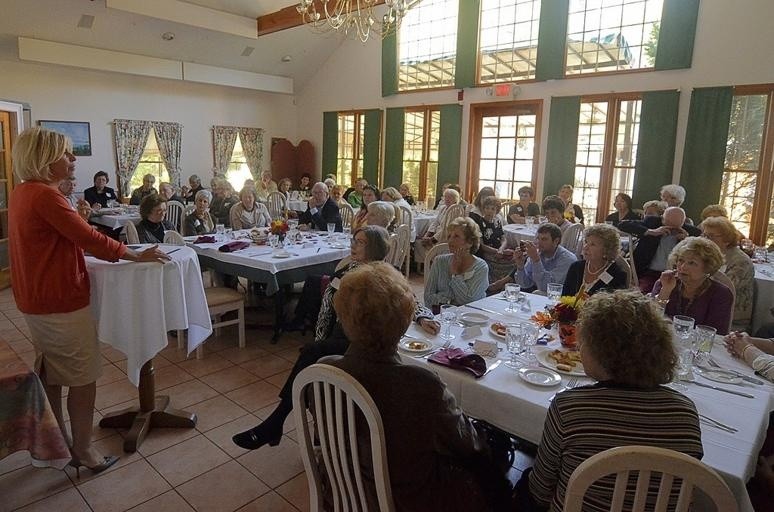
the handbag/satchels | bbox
[471,419,515,473]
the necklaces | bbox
[587,261,608,275]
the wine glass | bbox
[520,322,539,360]
[286,189,311,201]
[603,219,613,226]
[502,326,525,370]
[502,283,521,314]
[545,282,563,305]
[439,304,458,341]
[522,213,549,233]
[215,217,351,252]
[408,199,429,217]
[103,197,128,217]
[737,238,765,270]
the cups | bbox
[692,323,717,354]
[670,315,695,340]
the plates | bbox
[397,337,434,352]
[460,312,490,324]
[696,367,744,385]
[537,348,588,377]
[490,321,522,337]
[518,365,562,387]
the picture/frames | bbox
[38,120,92,156]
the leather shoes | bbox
[232,427,281,450]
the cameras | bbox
[520,240,526,252]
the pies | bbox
[548,349,582,372]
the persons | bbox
[423,217,488,315]
[508,288,704,511]
[302,261,513,512]
[230,171,291,297]
[652,236,736,336]
[7,127,172,479]
[701,204,754,324]
[607,184,701,291]
[421,183,468,244]
[470,185,584,296]
[288,173,415,337]
[231,226,440,450]
[559,222,629,298]
[181,174,238,292]
[723,331,773,493]
[64,171,183,246]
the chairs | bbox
[562,445,739,511]
[292,364,394,512]
[76,185,758,359]
[365,288,774,493]
[1,335,72,471]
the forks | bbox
[707,357,765,385]
[695,365,761,384]
[549,377,582,400]
[416,341,457,359]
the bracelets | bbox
[654,296,670,304]
[742,344,753,359]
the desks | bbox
[84,204,143,243]
[79,246,200,452]
[257,195,313,212]
[181,224,356,342]
[501,224,638,257]
[337,207,437,236]
[749,251,773,333]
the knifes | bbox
[464,303,503,316]
[679,379,753,400]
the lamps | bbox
[296,0,423,43]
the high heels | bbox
[69,454,120,478]
